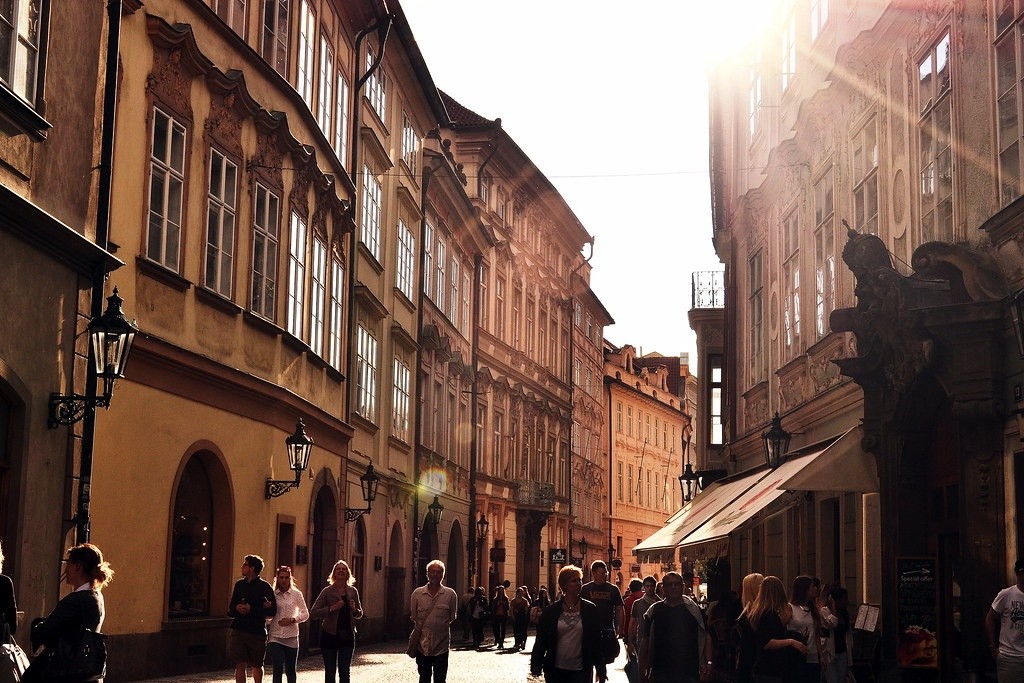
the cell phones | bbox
[263,597,269,602]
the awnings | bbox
[632,422,877,557]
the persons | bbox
[30,544,114,683]
[459,585,550,652]
[985,559,1024,683]
[530,566,606,683]
[0,537,18,644]
[614,572,851,683]
[264,566,309,682]
[581,560,625,678]
[310,561,364,683]
[410,560,457,683]
[230,555,276,683]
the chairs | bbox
[708,619,735,676]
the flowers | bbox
[694,560,722,580]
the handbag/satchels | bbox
[58,591,108,682]
[0,623,31,683]
[624,654,639,683]
[723,621,758,681]
[600,628,621,664]
[459,603,466,616]
[529,606,538,621]
[817,638,832,672]
[784,627,810,664]
[405,624,422,658]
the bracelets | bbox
[291,619,292,621]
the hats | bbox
[1015,560,1024,572]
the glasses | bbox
[60,558,77,564]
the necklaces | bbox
[708,661,712,665]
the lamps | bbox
[466,513,489,549]
[47,286,139,429]
[345,462,380,522]
[759,410,806,468]
[571,536,587,561]
[416,495,444,540]
[265,417,315,499]
[678,464,727,502]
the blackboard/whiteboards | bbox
[895,556,941,670]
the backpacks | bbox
[495,603,506,617]
